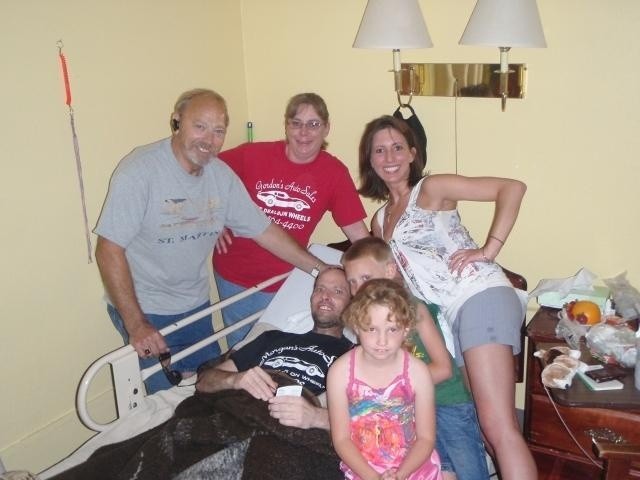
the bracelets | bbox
[487,232,507,245]
[481,250,496,264]
[310,261,325,276]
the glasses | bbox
[159,343,205,387]
[286,118,326,129]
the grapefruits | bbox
[571,300,601,325]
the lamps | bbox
[351,0,434,107]
[459,0,546,111]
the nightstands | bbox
[521,306,640,479]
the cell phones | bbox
[585,367,627,384]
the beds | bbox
[45,232,527,480]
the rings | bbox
[143,348,152,355]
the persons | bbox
[325,277,447,479]
[209,92,369,350]
[339,235,493,480]
[91,89,333,396]
[54,266,353,480]
[354,115,541,480]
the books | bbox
[576,362,626,395]
